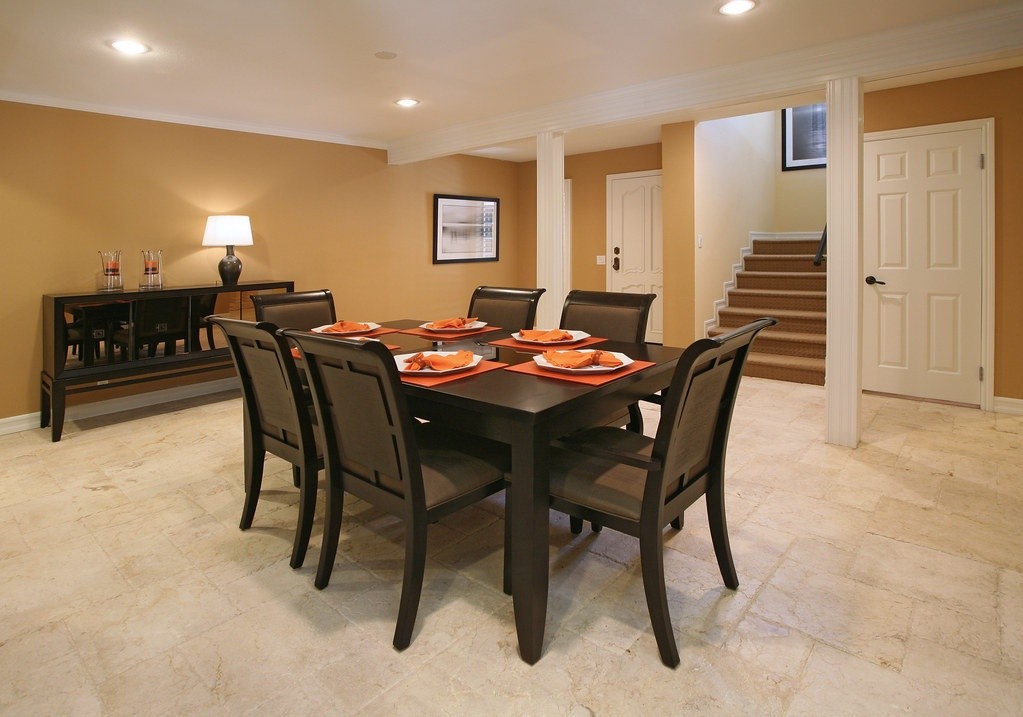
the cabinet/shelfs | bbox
[39,279,294,443]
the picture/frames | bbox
[781,103,826,171]
[433,193,500,264]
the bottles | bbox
[97,250,124,292]
[138,249,163,289]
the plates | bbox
[532,349,636,375]
[419,321,488,333]
[310,321,382,334]
[392,351,483,376]
[344,337,381,343]
[510,329,592,344]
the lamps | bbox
[201,215,253,286]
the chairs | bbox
[204,315,324,569]
[276,328,513,652]
[559,290,657,530]
[503,317,777,668]
[249,289,337,488]
[467,286,546,329]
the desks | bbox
[303,319,685,665]
